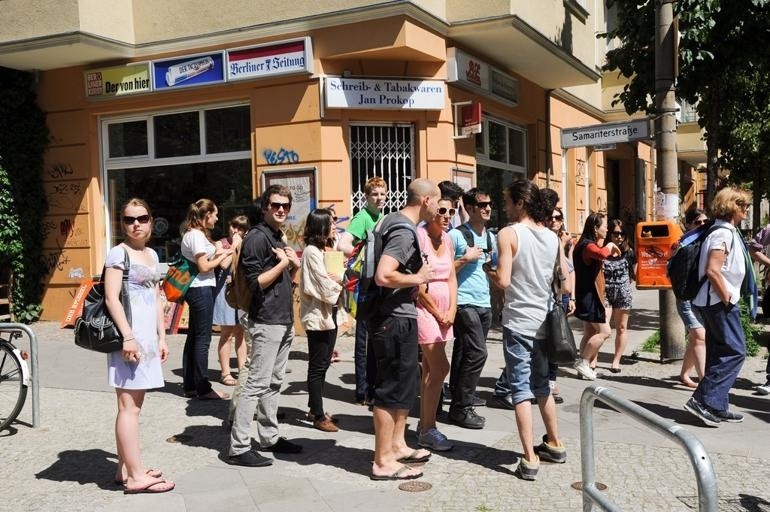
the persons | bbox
[590,219,636,372]
[365,178,441,481]
[434,180,466,403]
[337,176,389,406]
[103,197,175,492]
[445,187,499,429]
[486,179,577,481]
[227,183,302,466]
[326,209,343,364]
[211,214,249,387]
[572,212,622,380]
[677,211,713,389]
[171,198,230,403]
[299,208,346,432]
[750,224,770,396]
[411,198,458,451]
[683,187,750,426]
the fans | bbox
[153,217,168,237]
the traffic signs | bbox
[559,116,652,150]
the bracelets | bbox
[124,338,135,345]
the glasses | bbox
[547,213,562,223]
[470,201,494,209]
[437,207,457,219]
[267,198,291,213]
[330,214,338,221]
[736,201,751,212]
[610,230,626,238]
[123,214,150,225]
[690,218,710,225]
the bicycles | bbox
[0,324,32,432]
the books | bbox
[322,251,345,284]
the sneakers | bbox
[530,395,564,404]
[677,375,743,428]
[449,393,515,428]
[261,437,302,453]
[518,452,540,480]
[416,421,456,451]
[308,409,339,432]
[534,434,568,463]
[755,385,770,394]
[572,358,621,381]
[356,396,374,404]
[184,388,230,399]
[229,449,273,466]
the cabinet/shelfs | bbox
[218,204,252,237]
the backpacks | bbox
[225,224,278,313]
[669,225,734,300]
[341,214,423,321]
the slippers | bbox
[115,469,175,493]
[370,465,422,480]
[398,448,431,462]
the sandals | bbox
[220,374,236,386]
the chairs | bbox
[165,241,181,263]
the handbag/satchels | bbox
[162,251,199,304]
[74,282,132,354]
[547,306,577,364]
